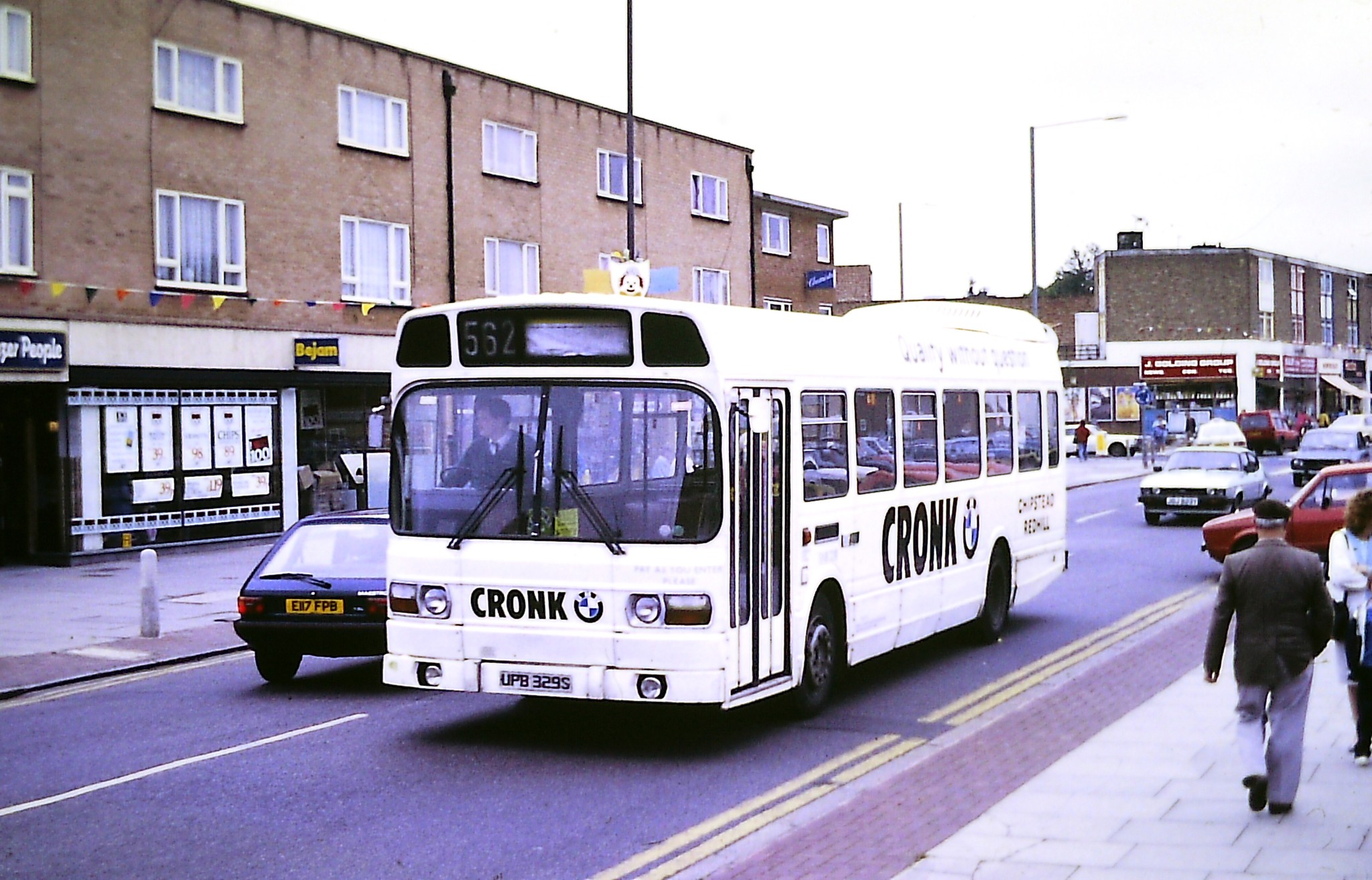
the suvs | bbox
[1236,408,1301,456]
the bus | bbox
[368,300,1071,720]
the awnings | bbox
[1322,373,1372,399]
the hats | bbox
[1251,500,1291,519]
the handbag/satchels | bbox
[1073,434,1077,443]
[1326,529,1348,642]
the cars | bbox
[1328,414,1372,446]
[232,507,391,685]
[1063,424,1151,458]
[771,426,1059,501]
[1137,418,1272,525]
[1289,427,1372,487]
[1200,462,1372,565]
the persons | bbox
[1337,406,1361,418]
[1073,420,1090,463]
[608,445,693,482]
[1294,409,1312,434]
[436,398,538,492]
[735,430,782,471]
[122,478,158,543]
[1316,406,1329,428]
[1183,413,1197,447]
[1204,502,1333,817]
[1327,490,1372,765]
[1152,416,1167,453]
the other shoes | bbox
[1269,804,1292,815]
[1248,779,1269,811]
[1354,756,1371,766]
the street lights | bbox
[1030,114,1129,318]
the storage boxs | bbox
[298,465,340,493]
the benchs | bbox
[559,480,721,539]
